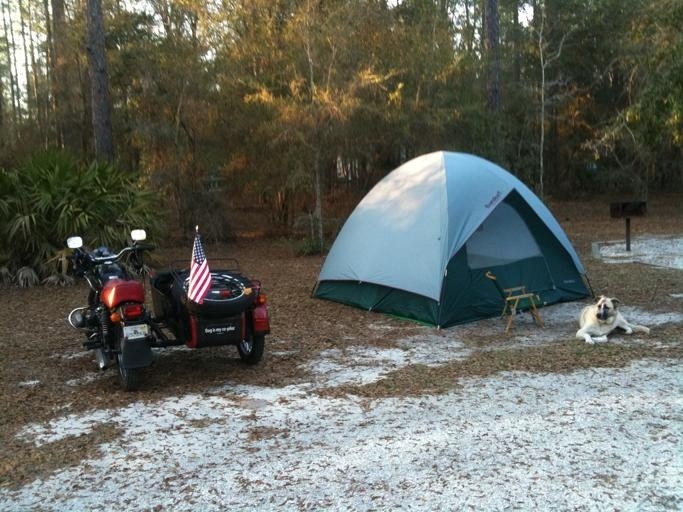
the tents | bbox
[311,149,595,330]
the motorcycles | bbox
[66,228,157,390]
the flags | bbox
[187,234,213,304]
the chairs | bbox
[486,272,545,335]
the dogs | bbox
[575,294,650,345]
